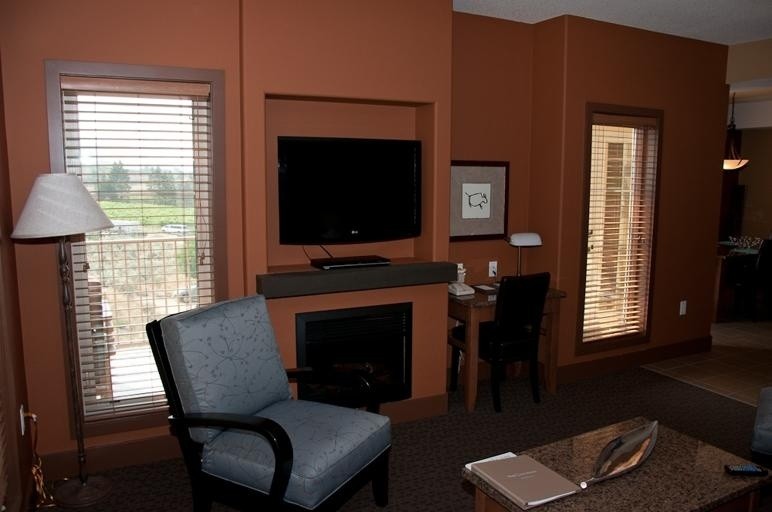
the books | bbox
[469,454,581,511]
[464,450,517,471]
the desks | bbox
[460,415,772,512]
[448,284,567,412]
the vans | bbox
[162,225,187,237]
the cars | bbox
[173,284,196,302]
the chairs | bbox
[450,272,551,412]
[145,294,393,511]
[749,386,771,507]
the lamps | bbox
[722,92,751,171]
[9,172,116,508]
[509,232,544,276]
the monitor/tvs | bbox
[277,135,423,244]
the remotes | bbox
[724,463,768,476]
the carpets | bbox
[33,367,757,512]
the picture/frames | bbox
[449,160,509,242]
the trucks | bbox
[112,221,148,236]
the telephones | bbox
[448,283,475,295]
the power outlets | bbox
[19,405,26,435]
[489,261,498,278]
